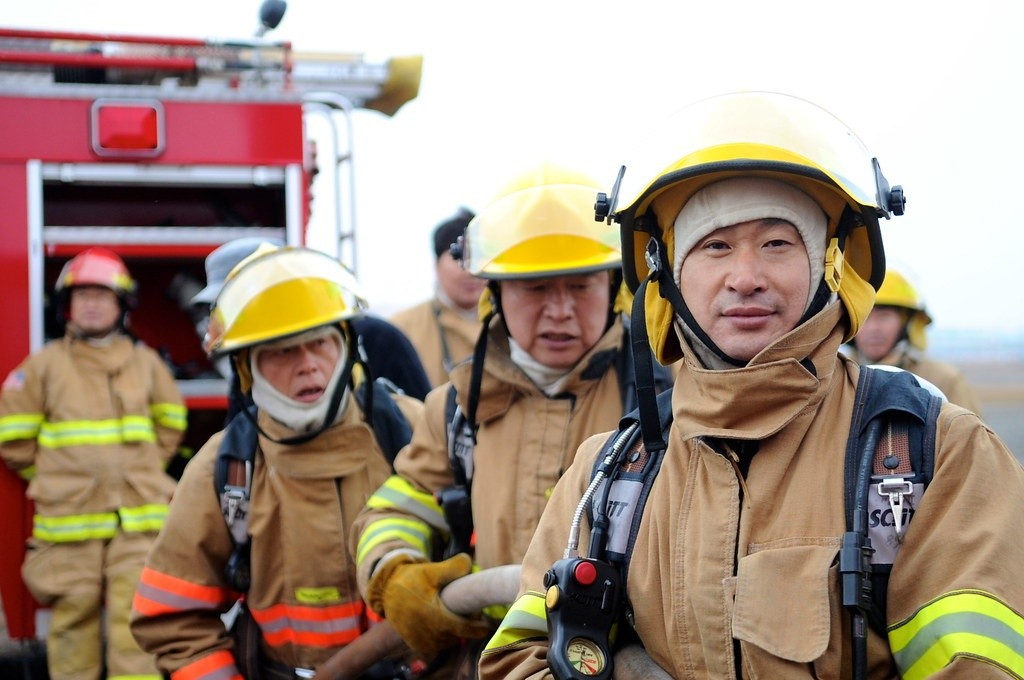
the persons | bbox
[129,245,425,680]
[847,272,978,415]
[387,205,488,392]
[479,92,1023,680]
[1,246,187,680]
[348,169,680,679]
[186,237,433,424]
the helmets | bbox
[55,246,138,312]
[875,267,933,353]
[594,91,941,366]
[448,164,635,323]
[204,241,368,397]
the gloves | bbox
[367,548,491,663]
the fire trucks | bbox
[0,25,424,658]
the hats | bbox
[189,236,286,316]
[434,207,475,258]
